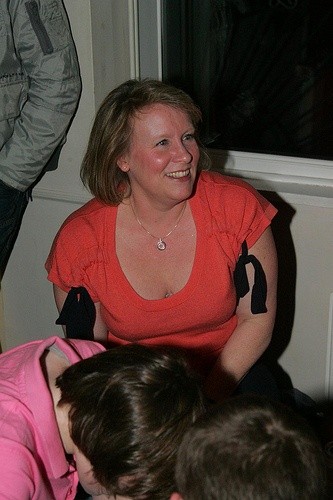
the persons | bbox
[0,335,213,500]
[169,401,333,500]
[51,78,278,384]
[0,0,82,292]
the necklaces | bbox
[126,194,190,251]
